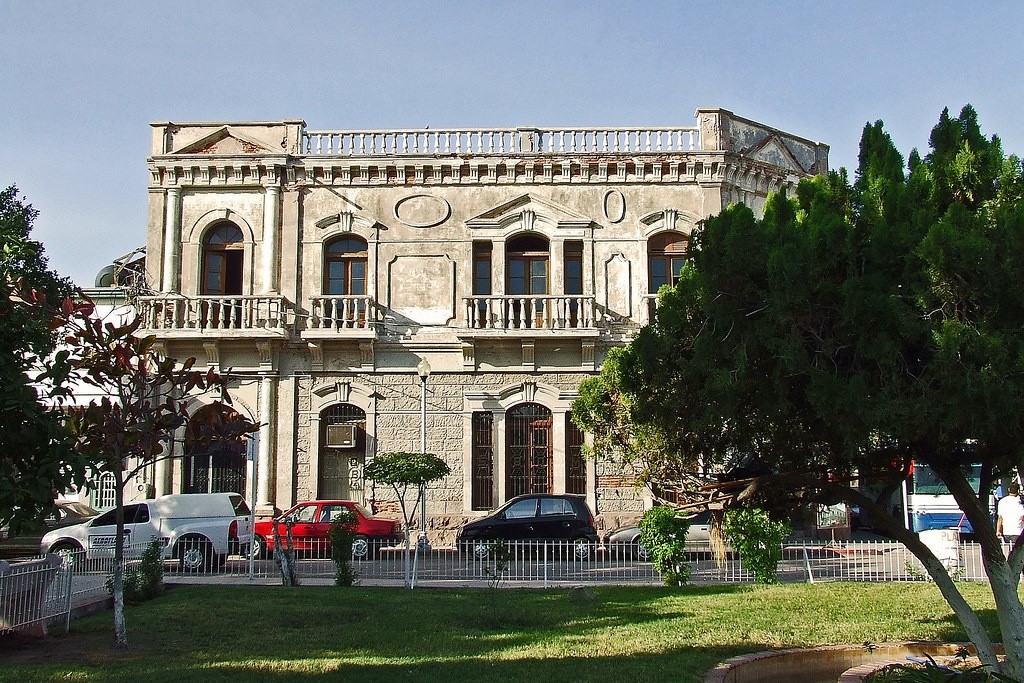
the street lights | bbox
[417,356,432,551]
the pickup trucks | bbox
[39,492,253,574]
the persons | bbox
[993,478,1024,543]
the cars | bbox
[602,508,766,562]
[245,500,405,560]
[34,500,101,531]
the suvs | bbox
[456,493,601,561]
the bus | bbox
[859,442,996,537]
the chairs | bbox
[320,507,330,522]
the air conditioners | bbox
[326,425,357,449]
